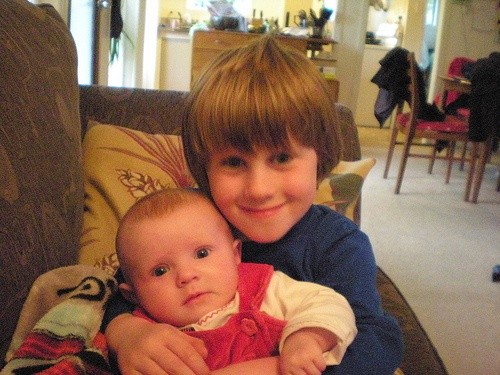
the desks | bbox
[438,74,500,203]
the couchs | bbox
[0,0,449,375]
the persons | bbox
[111,188,358,374]
[101,35,405,375]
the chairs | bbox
[383,51,480,202]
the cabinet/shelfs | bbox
[354,44,393,128]
[158,30,341,109]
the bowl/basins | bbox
[210,15,242,31]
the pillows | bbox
[78,121,377,269]
